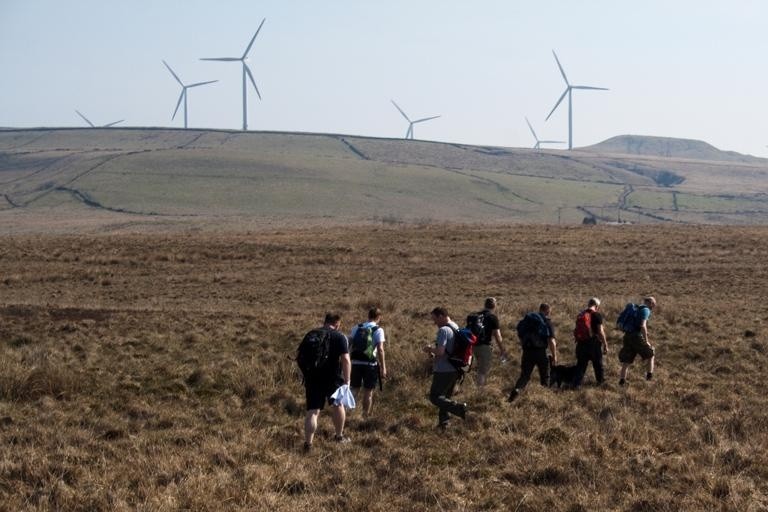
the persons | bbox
[420,305,468,429]
[573,296,611,387]
[465,296,509,386]
[295,312,353,453]
[508,302,558,402]
[617,296,658,385]
[347,307,387,418]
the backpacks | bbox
[466,311,486,344]
[349,322,380,362]
[515,311,548,352]
[435,324,476,369]
[574,310,597,343]
[616,302,648,334]
[295,327,336,376]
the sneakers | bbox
[455,403,466,420]
[302,436,351,457]
[507,389,521,403]
[647,372,658,381]
[619,378,630,385]
